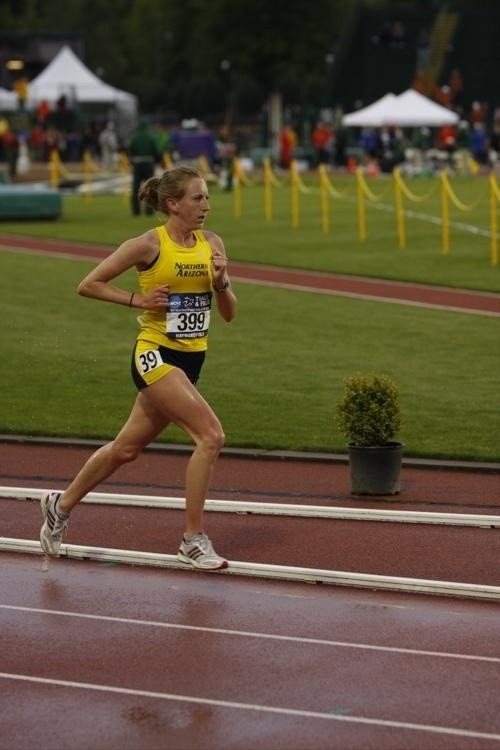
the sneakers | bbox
[178,534,229,570]
[40,489,70,557]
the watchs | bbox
[213,279,229,292]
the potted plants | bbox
[332,372,406,496]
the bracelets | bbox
[129,291,135,307]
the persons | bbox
[2,94,120,182]
[220,122,494,190]
[39,166,238,570]
[454,100,499,132]
[431,85,452,106]
[124,115,224,218]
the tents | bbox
[1,45,138,130]
[341,88,459,127]
